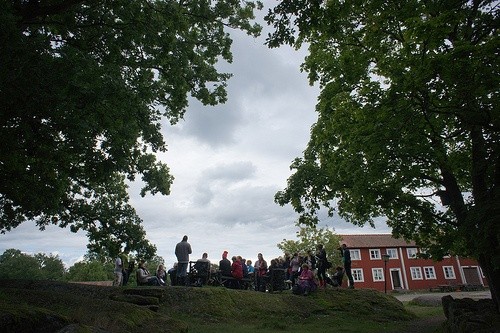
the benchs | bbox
[428,287,443,292]
[219,276,252,290]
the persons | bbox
[175,235,192,286]
[292,243,328,295]
[155,265,167,286]
[254,253,268,293]
[335,243,355,289]
[169,262,179,287]
[269,253,301,293]
[195,252,210,283]
[122,257,135,287]
[230,255,254,289]
[218,251,232,277]
[136,263,162,286]
[326,266,343,288]
[113,254,122,286]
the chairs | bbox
[135,271,147,285]
[262,267,286,291]
[187,261,211,287]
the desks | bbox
[436,284,451,293]
